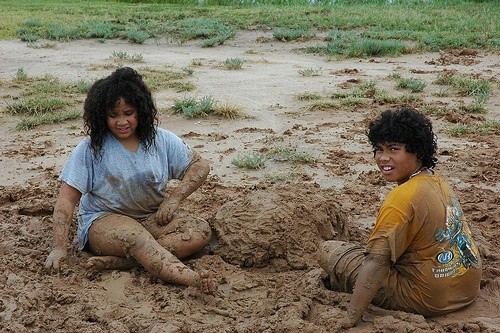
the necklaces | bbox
[409,165,428,179]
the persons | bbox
[41,66,217,295]
[316,104,482,331]
